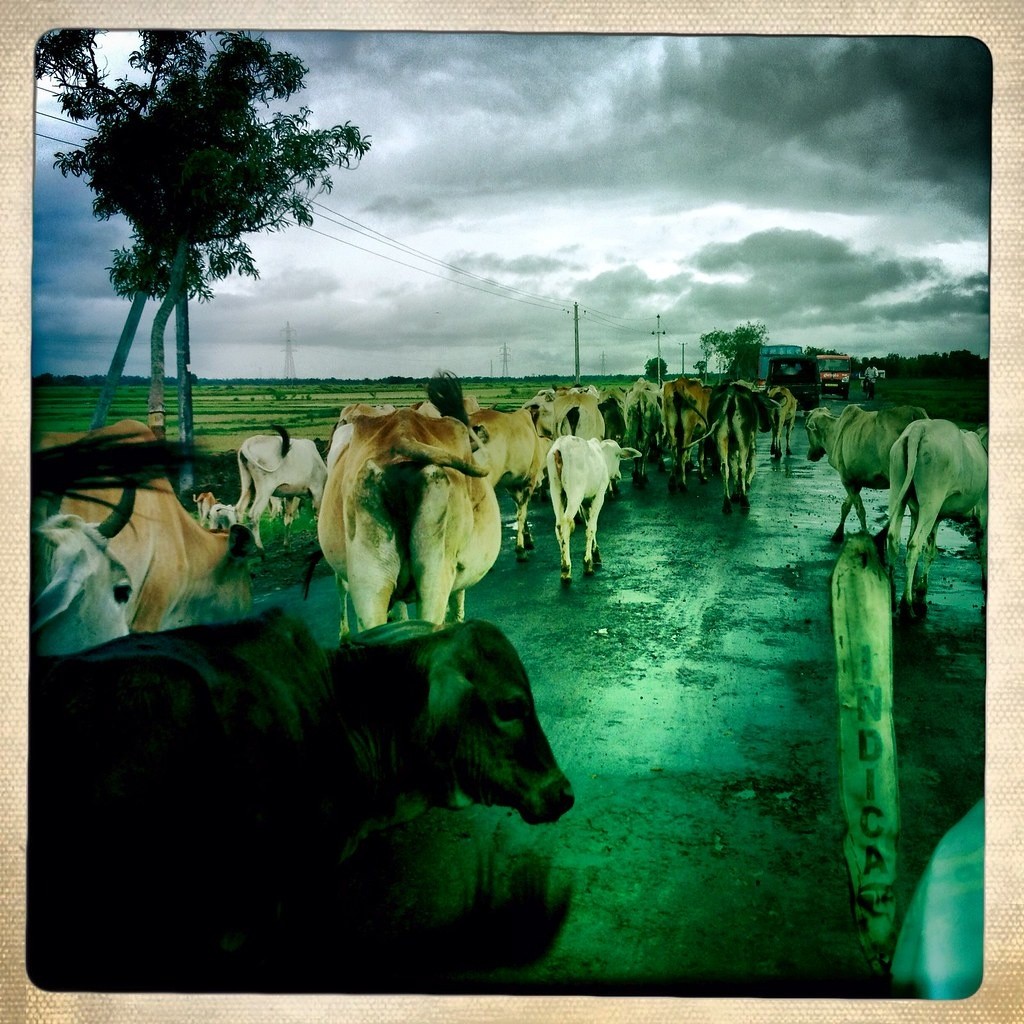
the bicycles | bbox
[863,375,879,402]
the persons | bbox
[864,363,879,400]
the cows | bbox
[27,620,572,995]
[31,420,260,663]
[190,376,800,632]
[798,400,987,619]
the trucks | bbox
[767,353,822,407]
[757,344,805,391]
[817,355,851,400]
